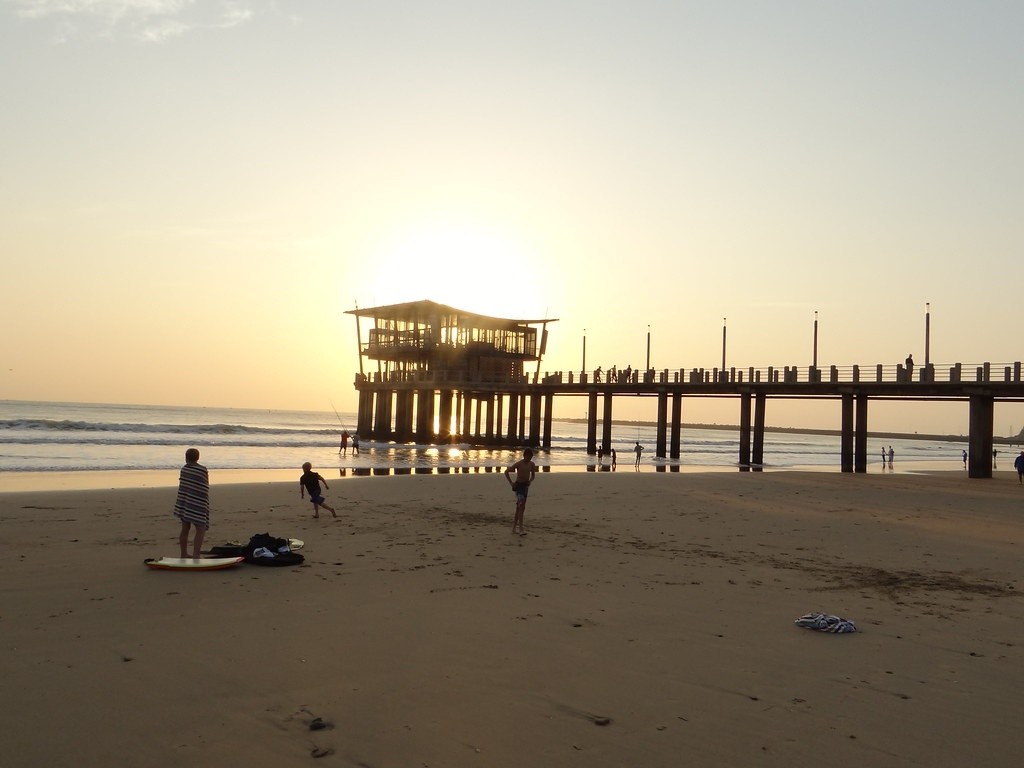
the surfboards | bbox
[143,556,245,571]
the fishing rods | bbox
[330,401,353,439]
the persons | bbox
[905,354,914,381]
[962,450,967,468]
[173,448,210,559]
[594,364,656,385]
[595,446,604,471]
[611,449,617,468]
[634,442,644,467]
[504,448,536,535]
[351,432,360,456]
[300,462,337,518]
[1015,451,1024,485]
[339,431,350,457]
[881,446,894,463]
